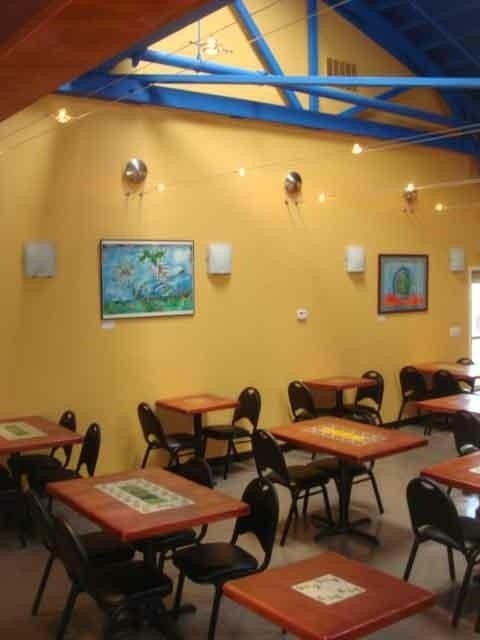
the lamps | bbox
[202,38,233,58]
[52,103,73,124]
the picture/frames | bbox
[378,252,428,315]
[99,237,197,321]
